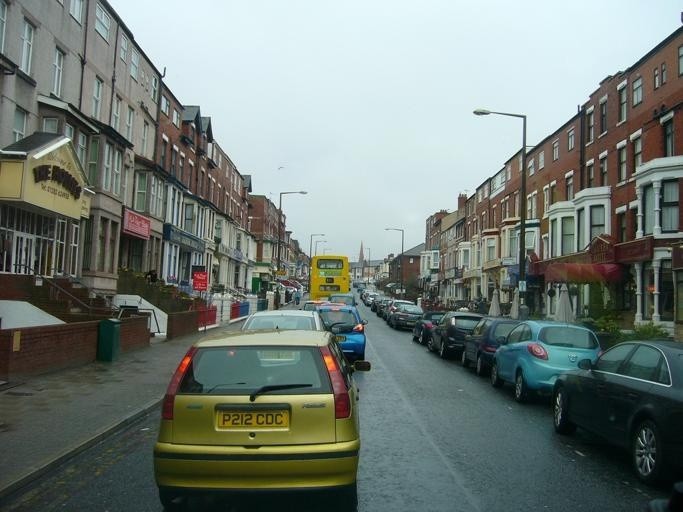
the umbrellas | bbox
[488,288,502,317]
[509,287,520,320]
[553,283,575,323]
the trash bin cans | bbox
[96,318,122,363]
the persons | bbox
[412,316,427,344]
[294,288,301,305]
[145,269,158,283]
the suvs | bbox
[151,326,370,512]
[427,310,485,362]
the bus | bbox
[308,255,351,303]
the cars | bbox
[270,278,301,293]
[227,310,329,367]
[302,293,368,362]
[460,317,523,376]
[552,339,683,491]
[352,279,444,346]
[491,320,601,405]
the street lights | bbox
[276,191,307,271]
[309,233,333,256]
[384,227,407,295]
[472,110,527,308]
[365,246,371,283]
